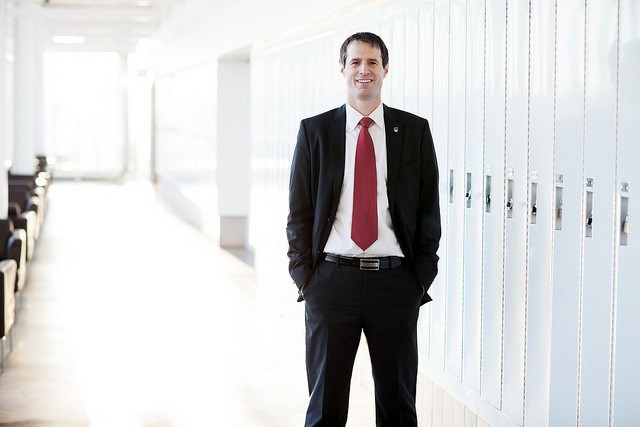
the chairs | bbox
[16,210,36,262]
[1,258,18,373]
[29,155,53,246]
[5,229,28,313]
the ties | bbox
[351,119,378,251]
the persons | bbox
[286,31,442,426]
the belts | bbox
[325,254,406,272]
